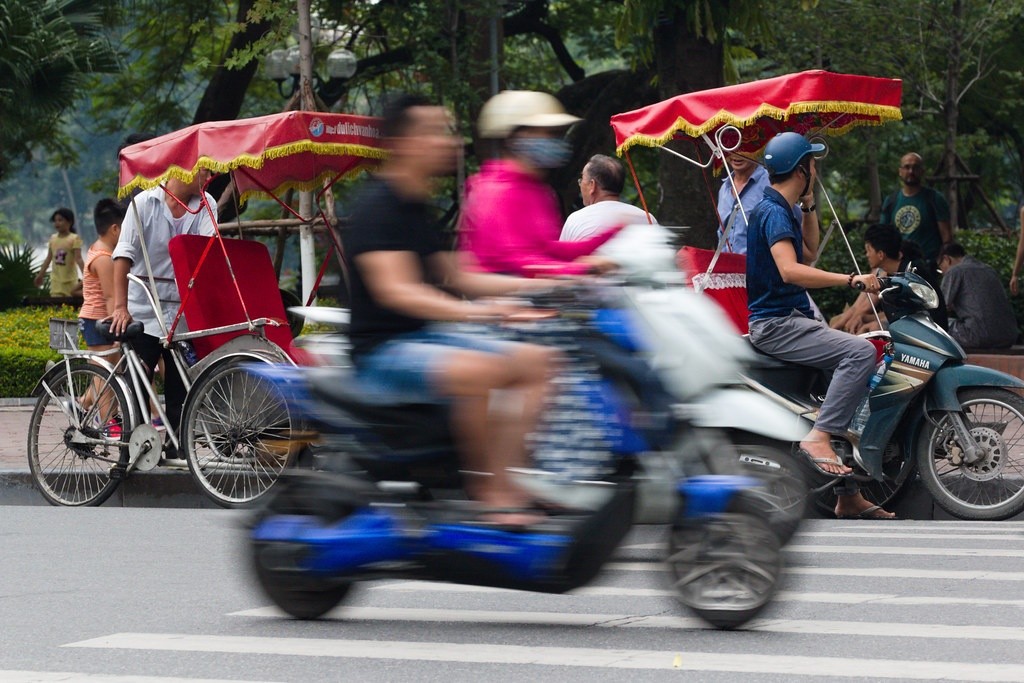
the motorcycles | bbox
[239,281,793,629]
[734,269,1023,522]
[289,227,809,545]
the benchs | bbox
[679,246,751,340]
[166,233,310,368]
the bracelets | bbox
[846,272,858,288]
[800,202,816,212]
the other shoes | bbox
[151,412,166,430]
[163,441,207,467]
[62,399,100,430]
[92,415,122,441]
[459,496,567,529]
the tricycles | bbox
[608,71,918,517]
[28,110,390,509]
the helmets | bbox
[474,89,586,139]
[762,131,826,176]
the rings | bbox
[871,284,874,286]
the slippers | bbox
[799,448,855,479]
[836,505,899,520]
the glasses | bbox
[578,177,592,186]
[899,164,921,170]
[936,257,944,270]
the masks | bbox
[500,137,574,170]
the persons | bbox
[34,208,85,297]
[878,153,951,264]
[830,222,1019,350]
[1009,206,1024,294]
[560,155,655,242]
[745,132,898,519]
[65,197,165,440]
[345,89,633,527]
[101,166,217,458]
[715,154,803,253]
